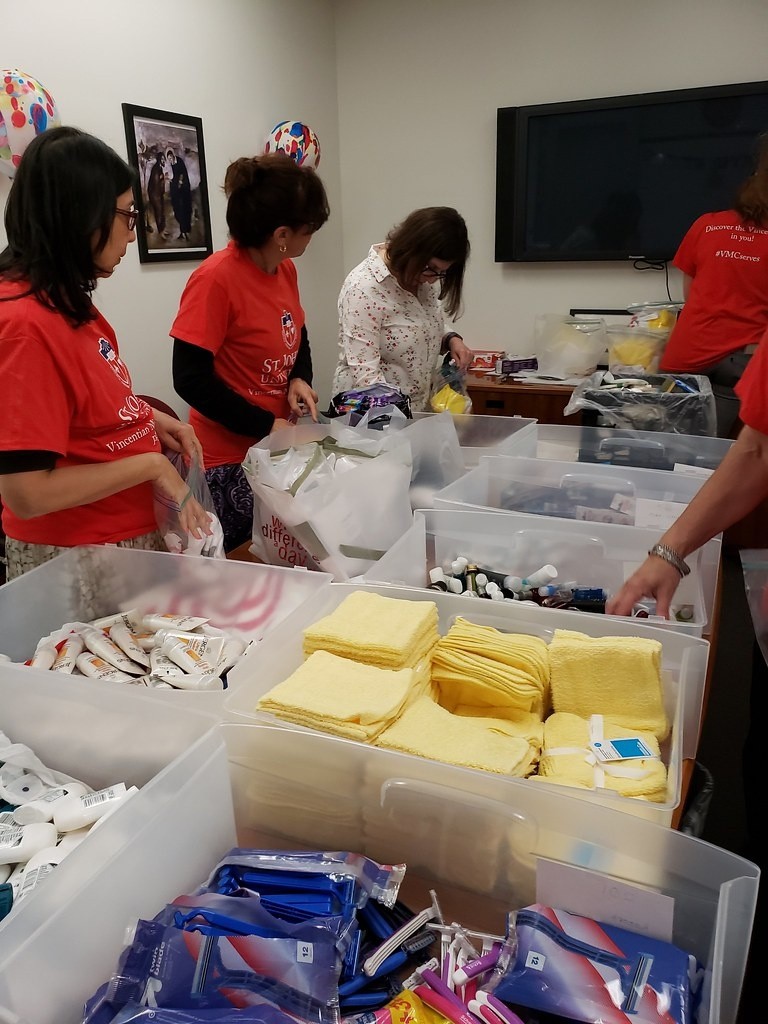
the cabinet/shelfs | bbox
[463,374,584,425]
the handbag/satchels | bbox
[625,301,684,334]
[429,352,473,415]
[536,312,612,379]
[241,404,467,583]
[153,443,226,559]
[603,324,669,375]
[327,383,413,431]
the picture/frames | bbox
[123,103,214,264]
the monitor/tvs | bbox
[495,82,768,263]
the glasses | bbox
[115,208,139,231]
[421,265,446,281]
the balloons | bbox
[0,66,56,178]
[263,119,321,171]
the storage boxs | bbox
[0,412,761,1024]
[468,349,502,372]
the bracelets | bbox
[648,543,691,577]
[445,331,464,349]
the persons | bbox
[0,128,212,587]
[658,131,768,441]
[331,206,474,417]
[606,331,767,622]
[169,152,329,549]
